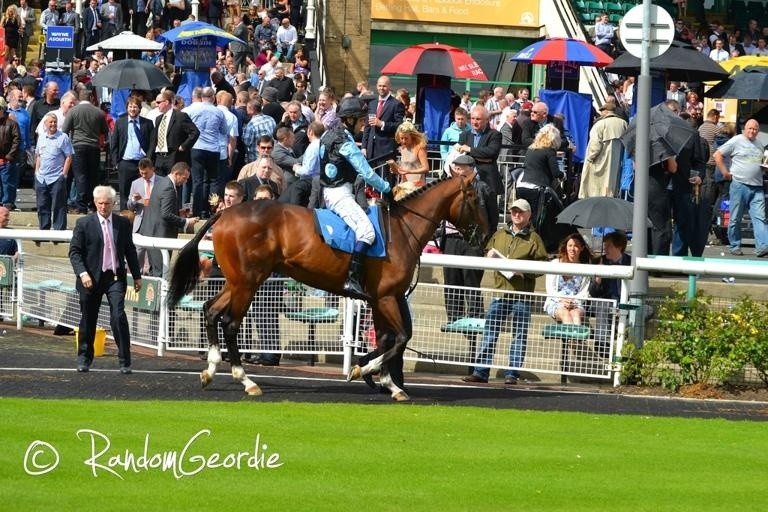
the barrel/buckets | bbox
[73,327,106,357]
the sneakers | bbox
[730,249,743,255]
[757,246,768,257]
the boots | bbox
[344,250,372,297]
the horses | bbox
[165,164,495,401]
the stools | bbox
[541,322,591,386]
[22,278,64,328]
[284,306,339,366]
[181,299,209,360]
[440,316,486,376]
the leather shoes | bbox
[441,321,460,332]
[120,367,131,374]
[505,375,517,384]
[461,373,488,383]
[77,364,88,372]
[222,352,279,366]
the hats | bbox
[598,102,616,111]
[508,199,531,212]
[452,155,475,165]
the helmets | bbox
[335,97,369,118]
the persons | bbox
[0,1,767,391]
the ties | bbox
[257,82,261,92]
[157,114,167,151]
[102,218,117,275]
[376,100,385,119]
[144,180,151,206]
[131,119,148,154]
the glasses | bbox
[156,100,165,105]
